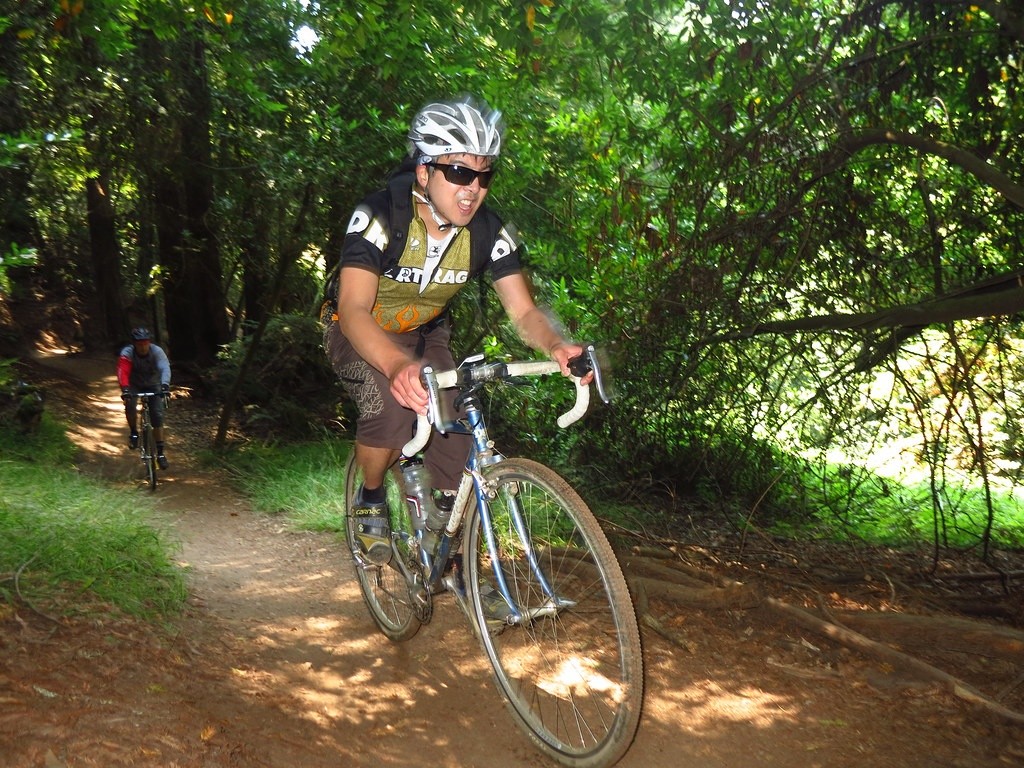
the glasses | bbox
[424,161,495,189]
[136,340,150,346]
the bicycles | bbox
[121,391,171,490]
[344,343,643,768]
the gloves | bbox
[121,389,134,401]
[159,385,170,398]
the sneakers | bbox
[453,566,512,619]
[157,453,170,470]
[128,432,139,450]
[351,483,392,565]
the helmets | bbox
[407,102,502,161]
[131,327,151,340]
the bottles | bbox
[400,455,433,531]
[420,489,455,555]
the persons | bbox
[319,99,595,625]
[116,327,171,470]
